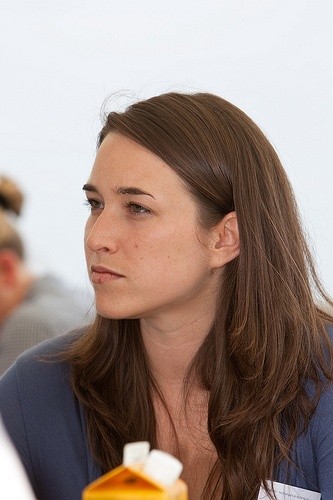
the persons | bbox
[0,173,98,375]
[0,87,333,500]
